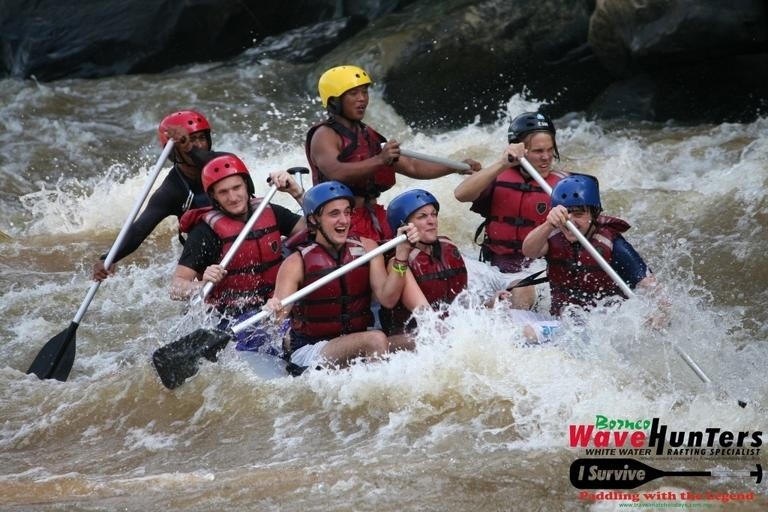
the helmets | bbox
[387,190,440,233]
[507,111,555,142]
[302,181,356,220]
[201,156,254,192]
[551,174,602,213]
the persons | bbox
[306,66,481,241]
[262,182,419,365]
[170,156,308,360]
[507,175,672,344]
[93,112,236,282]
[378,190,513,337]
[454,114,572,312]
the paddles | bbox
[151,230,417,393]
[24,136,175,384]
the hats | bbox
[318,65,372,109]
[160,111,211,148]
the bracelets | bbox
[294,189,305,199]
[392,258,409,278]
